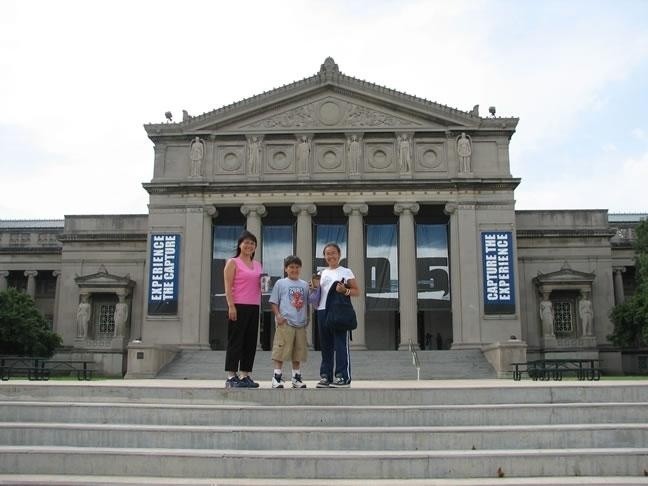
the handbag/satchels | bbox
[326,281,357,330]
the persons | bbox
[297,134,313,174]
[347,134,362,172]
[458,132,472,174]
[538,293,556,338]
[223,230,263,391]
[267,257,310,389]
[425,332,433,348]
[398,133,413,173]
[578,292,596,336]
[189,135,204,177]
[248,135,261,174]
[436,332,442,348]
[114,293,128,335]
[76,292,91,338]
[310,244,361,391]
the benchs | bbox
[0,355,101,386]
[505,355,605,383]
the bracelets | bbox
[342,286,351,298]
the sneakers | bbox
[329,378,351,387]
[316,377,333,388]
[272,372,285,388]
[226,375,259,387]
[292,373,306,388]
